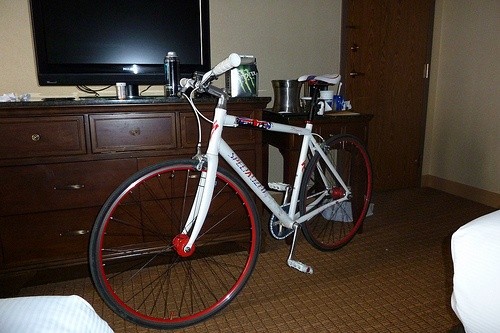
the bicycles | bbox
[88,52,373,329]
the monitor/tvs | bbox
[28,0,211,98]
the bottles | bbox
[164,51,178,97]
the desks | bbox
[262,107,376,246]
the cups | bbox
[271,79,304,113]
[320,91,333,109]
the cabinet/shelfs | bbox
[0,96,273,293]
